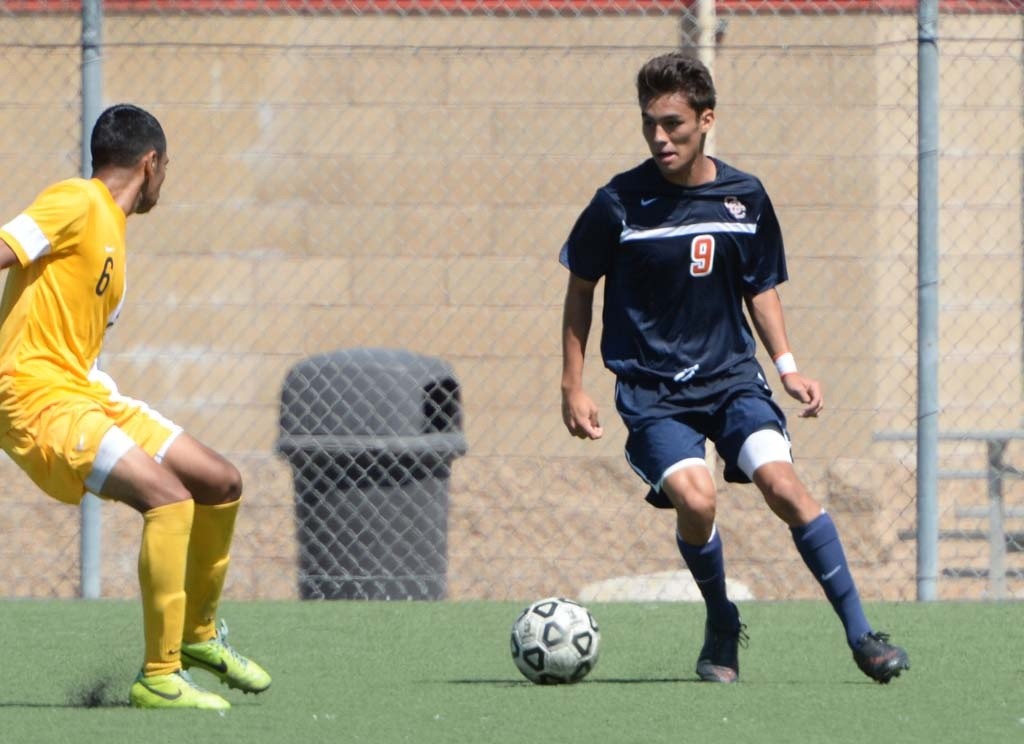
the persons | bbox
[0,104,270,708]
[557,53,909,685]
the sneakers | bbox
[852,631,911,683]
[695,602,748,682]
[127,666,229,710]
[182,618,271,694]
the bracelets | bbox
[772,350,797,381]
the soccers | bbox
[509,597,601,686]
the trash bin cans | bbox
[276,348,469,603]
